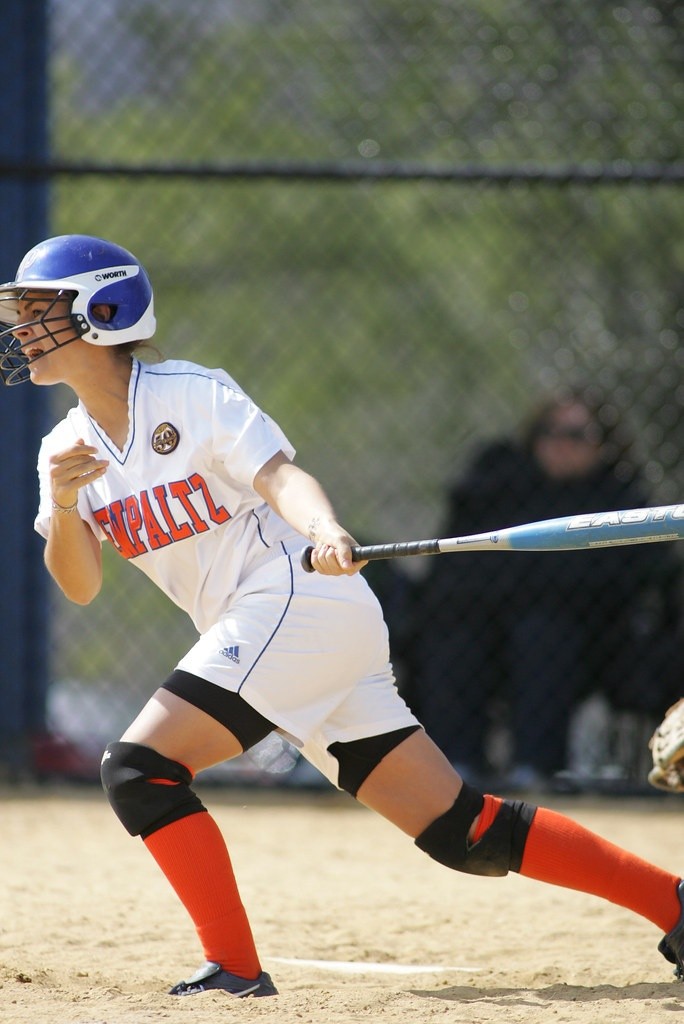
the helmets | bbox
[0,234,156,347]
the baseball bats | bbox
[299,500,684,575]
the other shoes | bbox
[657,880,684,981]
[167,961,280,998]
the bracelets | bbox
[51,497,79,514]
[308,516,336,545]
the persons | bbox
[406,384,672,794]
[0,232,683,995]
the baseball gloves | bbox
[646,695,684,796]
